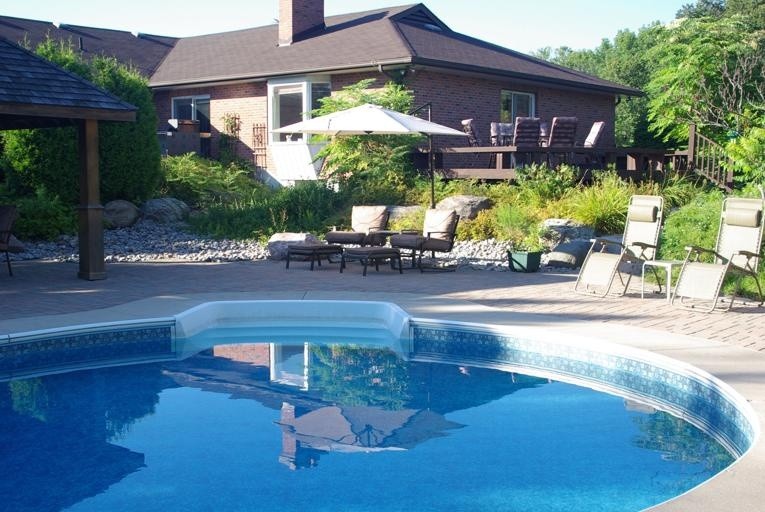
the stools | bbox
[286,243,402,276]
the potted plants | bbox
[494,202,544,272]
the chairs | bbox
[573,195,765,314]
[0,205,22,277]
[460,115,605,170]
[325,204,460,272]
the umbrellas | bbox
[270,100,471,259]
[271,363,471,453]
